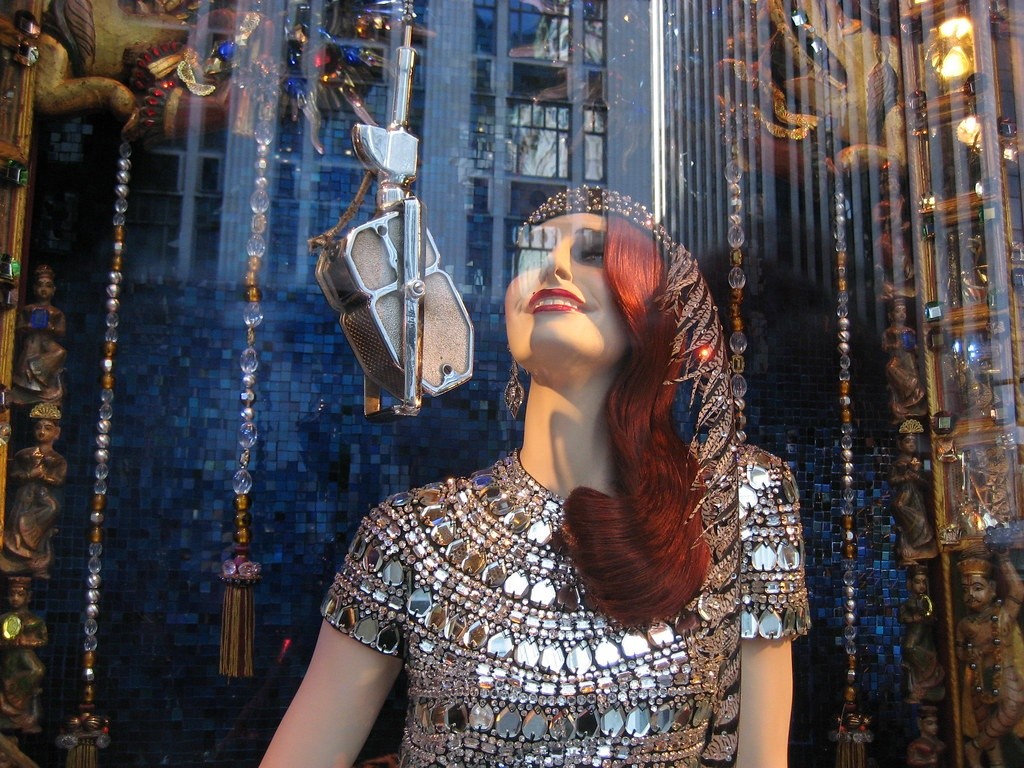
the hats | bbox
[515,185,679,260]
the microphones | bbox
[314,207,473,401]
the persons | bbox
[257,184,810,768]
[0,263,67,731]
[869,172,1024,768]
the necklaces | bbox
[966,599,1003,705]
